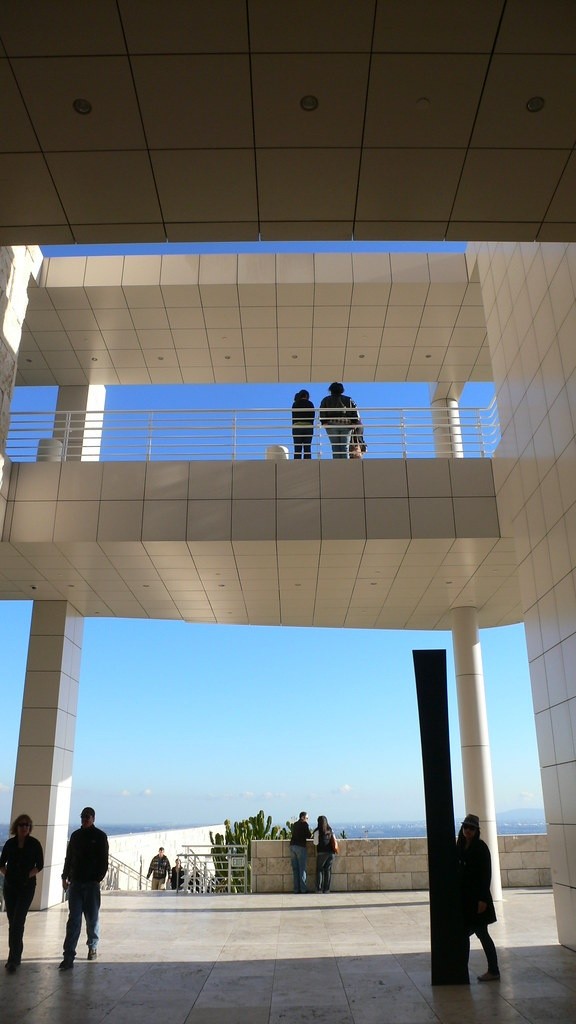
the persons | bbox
[289,812,312,893]
[319,382,359,459]
[313,816,333,893]
[147,847,172,890]
[171,858,185,890]
[0,815,44,969]
[291,390,315,459]
[456,813,500,981]
[188,867,204,893]
[57,807,110,968]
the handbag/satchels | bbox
[329,827,338,855]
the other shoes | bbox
[6,961,16,972]
[477,972,501,981]
[59,959,74,969]
[15,960,21,965]
[88,948,96,960]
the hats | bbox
[460,814,480,829]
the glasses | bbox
[81,813,91,818]
[462,824,476,831]
[17,822,30,828]
